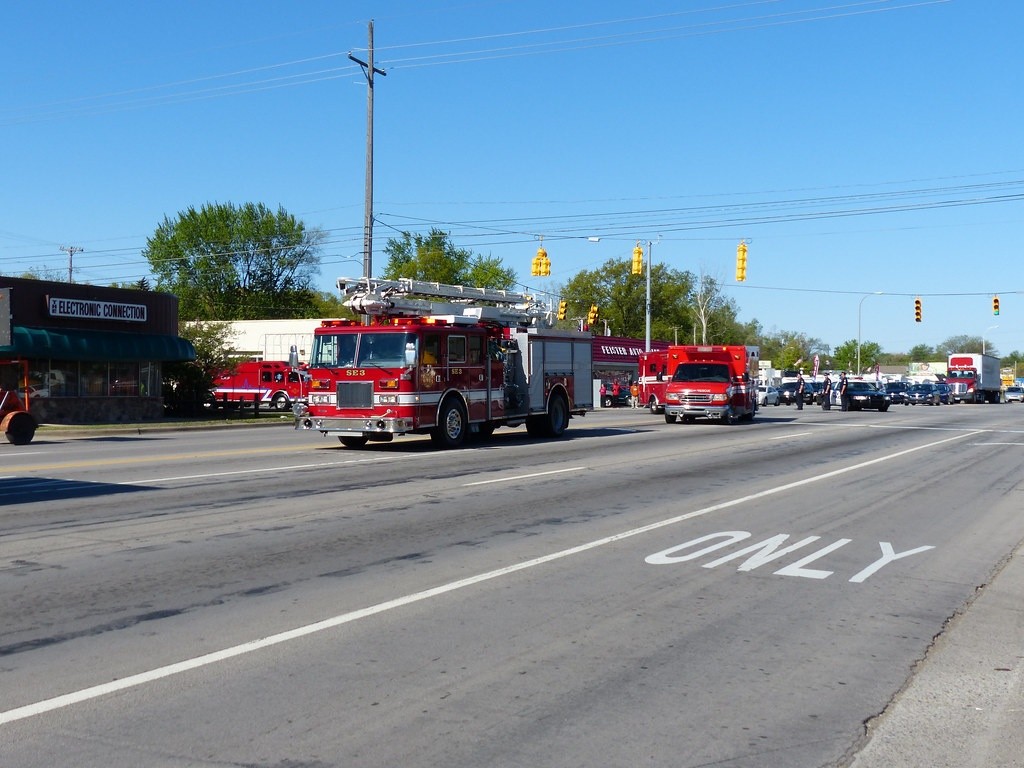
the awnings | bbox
[0,325,196,362]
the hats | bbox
[633,380,637,383]
[824,373,827,375]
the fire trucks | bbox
[638,344,760,424]
[208,361,312,413]
[289,277,595,451]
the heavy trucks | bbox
[945,353,1001,404]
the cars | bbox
[932,383,954,405]
[811,377,912,405]
[0,360,39,446]
[904,384,941,407]
[604,385,632,408]
[1003,387,1024,404]
[18,385,50,398]
[775,382,814,406]
[758,385,780,408]
[829,380,891,413]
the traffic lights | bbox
[588,311,596,325]
[993,297,999,315]
[526,296,532,306]
[531,259,541,276]
[558,299,567,320]
[541,260,551,276]
[590,305,599,326]
[914,299,921,323]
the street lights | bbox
[983,325,1000,355]
[858,292,883,376]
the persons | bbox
[840,372,848,412]
[822,373,831,410]
[422,348,437,364]
[630,381,639,409]
[612,379,620,409]
[599,383,606,408]
[795,373,804,410]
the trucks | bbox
[42,370,78,395]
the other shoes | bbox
[632,407,634,409]
[823,408,830,410]
[795,408,803,410]
[635,407,638,409]
[839,409,847,411]
